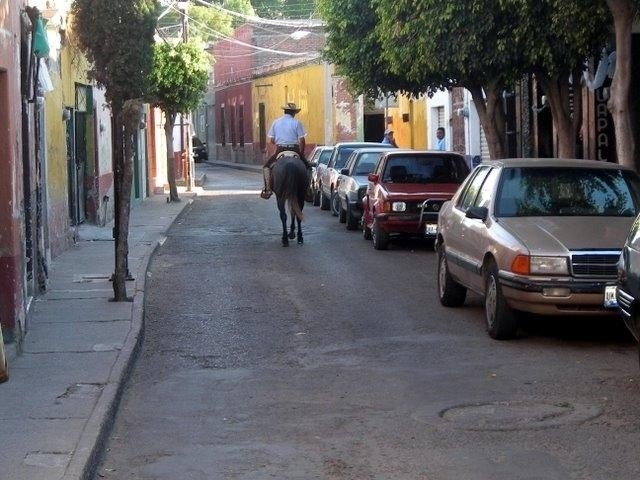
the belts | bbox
[278,143,296,147]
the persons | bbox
[435,127,445,151]
[261,103,313,199]
[381,129,399,148]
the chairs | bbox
[431,164,451,183]
[388,165,409,181]
[485,195,601,216]
[358,162,376,173]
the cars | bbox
[306,140,471,251]
[193,136,210,164]
[433,157,640,345]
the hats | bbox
[282,102,301,114]
[384,128,394,135]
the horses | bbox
[271,156,309,247]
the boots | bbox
[261,166,274,199]
